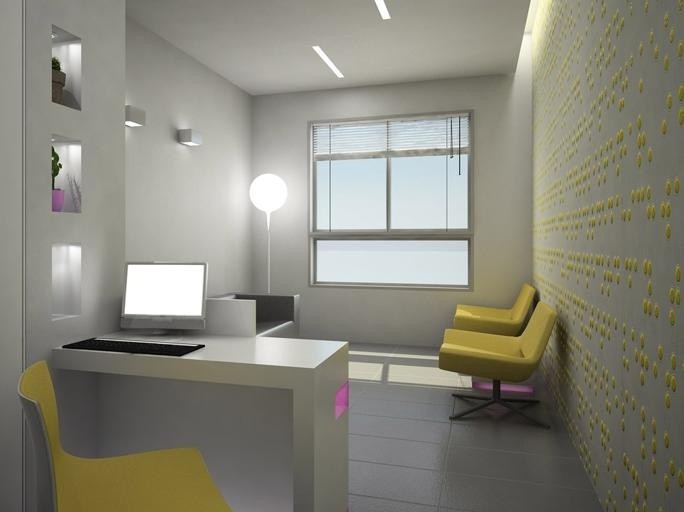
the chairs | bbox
[438,282,558,430]
[206,292,299,339]
[17,360,232,512]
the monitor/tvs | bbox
[117,262,208,341]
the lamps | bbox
[125,103,146,129]
[248,173,288,295]
[178,129,202,146]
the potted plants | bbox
[51,56,66,103]
[52,145,65,211]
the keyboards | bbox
[62,337,205,357]
